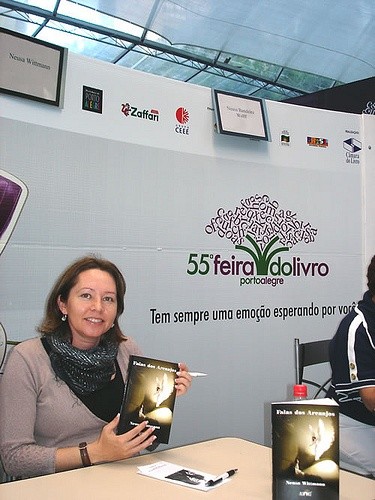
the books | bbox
[271,397,340,500]
[116,355,180,444]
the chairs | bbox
[294,338,333,401]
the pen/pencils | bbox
[205,468,238,486]
[189,371,208,379]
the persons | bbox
[0,257,192,484]
[325,254,375,480]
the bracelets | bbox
[79,441,94,468]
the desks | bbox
[1,436,375,500]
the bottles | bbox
[290,385,308,399]
